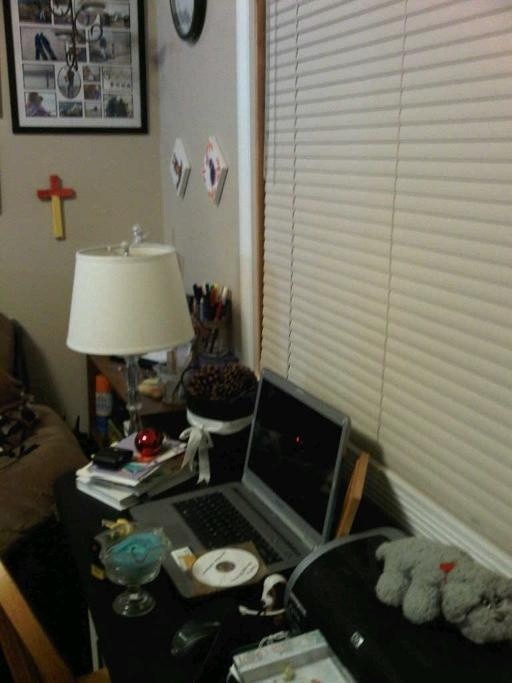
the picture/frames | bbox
[0,0,150,135]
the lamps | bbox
[66,241,197,433]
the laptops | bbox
[129,365,352,600]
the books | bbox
[74,423,199,513]
[231,626,359,683]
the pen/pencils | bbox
[186,281,229,353]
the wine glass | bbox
[94,521,172,617]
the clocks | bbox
[169,0,206,42]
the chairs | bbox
[0,559,110,683]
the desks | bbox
[85,355,186,447]
[54,472,512,683]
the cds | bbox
[191,546,260,588]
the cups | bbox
[192,318,229,359]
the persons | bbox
[97,35,108,61]
[35,6,48,23]
[27,91,48,117]
[63,70,76,98]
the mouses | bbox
[165,618,225,660]
